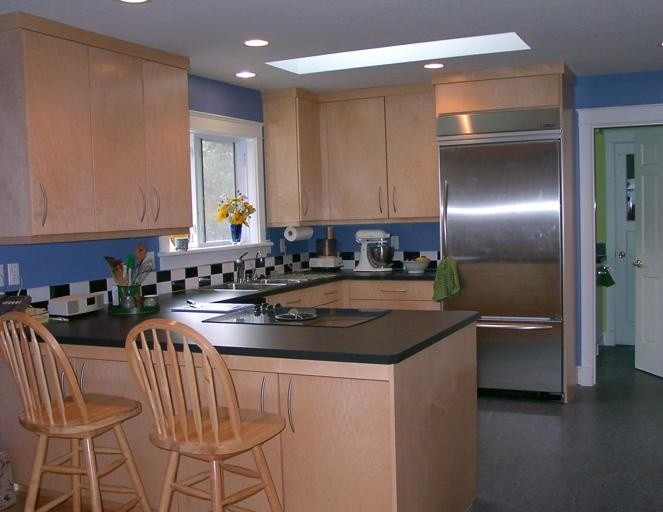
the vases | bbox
[171,237,189,251]
[231,224,242,245]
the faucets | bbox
[251,250,262,280]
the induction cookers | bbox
[202,303,393,328]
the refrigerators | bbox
[434,105,562,393]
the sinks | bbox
[200,282,286,292]
[246,278,312,284]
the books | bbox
[7,307,50,331]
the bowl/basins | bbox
[400,260,430,273]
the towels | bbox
[431,256,461,302]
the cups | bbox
[174,238,188,251]
[144,294,160,306]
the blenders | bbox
[353,228,395,272]
[309,226,343,272]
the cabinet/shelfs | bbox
[88,32,193,241]
[263,279,345,312]
[346,279,443,311]
[1,342,179,512]
[262,89,322,227]
[319,83,440,226]
[0,11,94,244]
[179,320,478,512]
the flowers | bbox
[216,190,256,228]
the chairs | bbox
[125,318,286,512]
[1,310,153,512]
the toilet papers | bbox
[284,227,314,242]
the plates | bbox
[274,313,318,321]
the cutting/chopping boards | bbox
[170,302,255,314]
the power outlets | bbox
[7,262,21,286]
